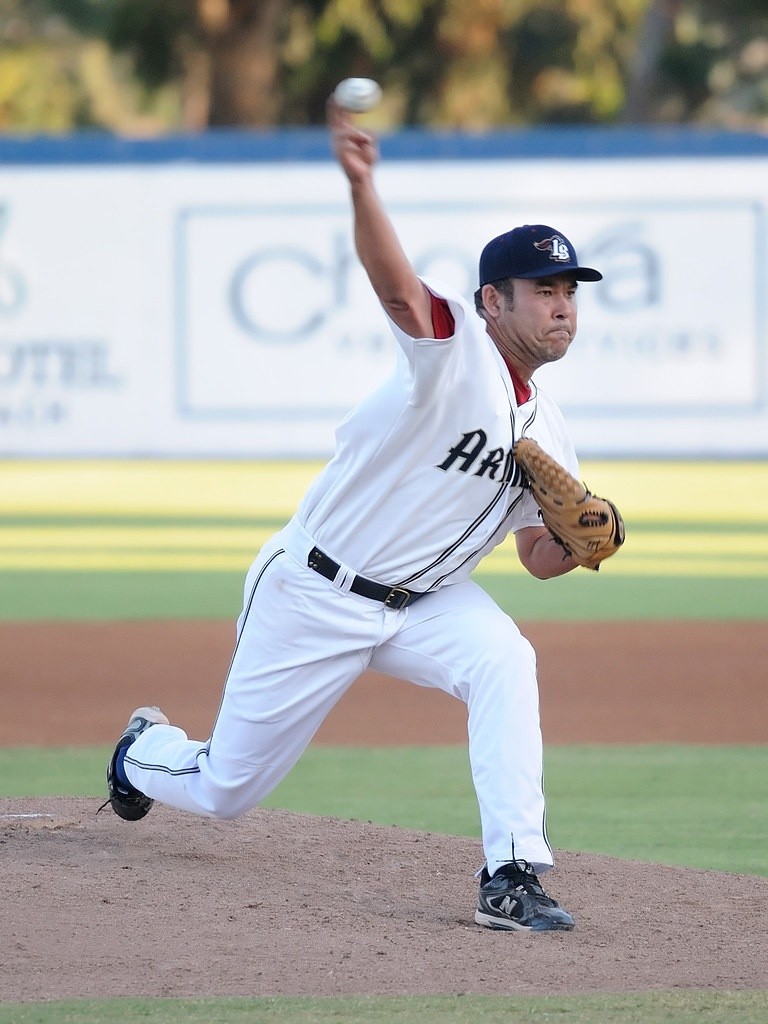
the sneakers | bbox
[474,833,574,932]
[95,707,170,821]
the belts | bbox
[309,545,437,611]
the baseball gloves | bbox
[509,433,628,570]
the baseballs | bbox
[332,75,383,116]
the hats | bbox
[480,225,602,286]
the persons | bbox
[107,97,628,931]
